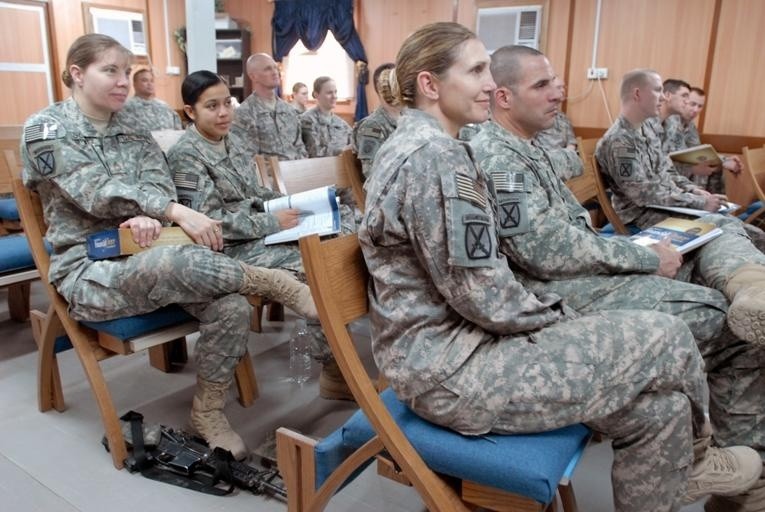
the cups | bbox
[235,76,243,86]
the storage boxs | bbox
[216,39,242,59]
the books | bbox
[619,216,723,257]
[670,144,724,167]
[264,184,341,245]
[646,201,742,217]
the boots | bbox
[190,375,248,462]
[237,259,320,321]
[722,265,764,348]
[319,358,388,402]
[674,435,763,509]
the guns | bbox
[103,411,288,503]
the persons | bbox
[595,68,765,251]
[473,45,765,361]
[668,88,745,193]
[226,53,402,179]
[652,78,717,191]
[540,74,580,152]
[21,33,322,464]
[124,68,182,129]
[167,70,378,401]
[355,22,765,512]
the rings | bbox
[213,228,220,233]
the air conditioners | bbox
[476,5,543,55]
[90,7,147,56]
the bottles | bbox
[290,318,311,386]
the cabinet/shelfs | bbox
[186,28,251,103]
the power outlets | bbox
[587,68,608,80]
